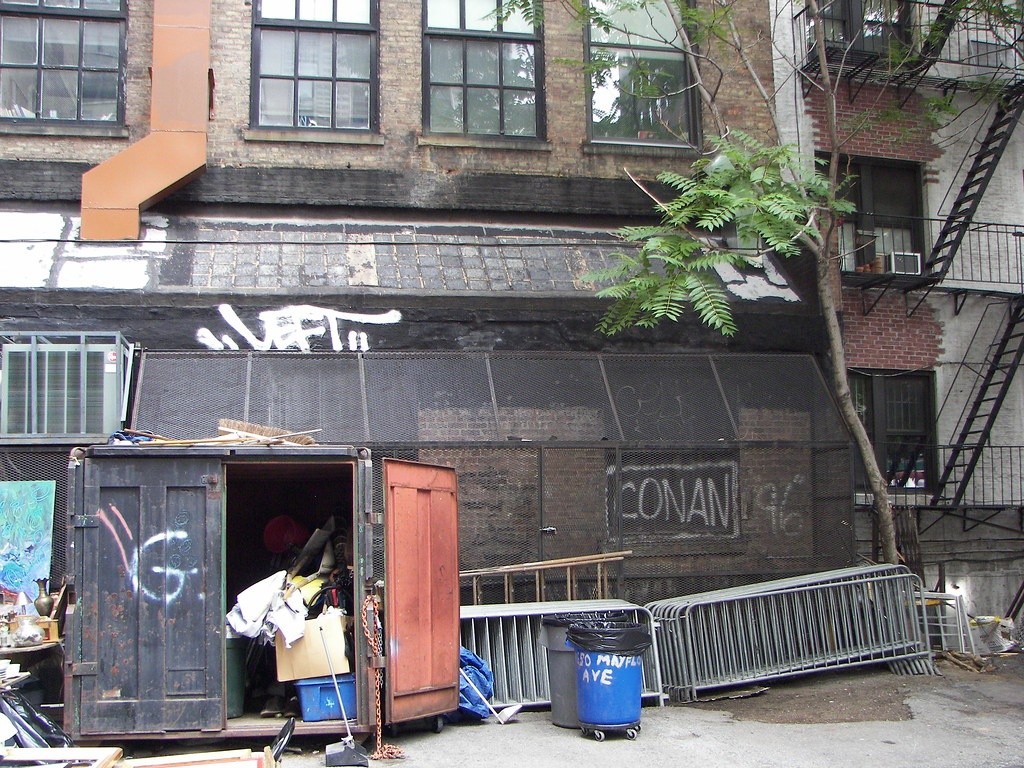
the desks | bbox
[0,640,61,747]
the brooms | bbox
[217,418,316,446]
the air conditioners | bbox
[1,343,130,444]
[887,252,921,275]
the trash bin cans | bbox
[565,620,652,741]
[228,621,246,721]
[540,612,631,729]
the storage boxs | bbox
[270,617,351,683]
[293,675,357,722]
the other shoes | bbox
[283,702,300,718]
[260,697,284,717]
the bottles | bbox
[11,615,45,646]
[33,577,54,620]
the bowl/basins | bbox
[6,664,20,678]
[0,660,10,669]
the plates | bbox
[0,668,7,679]
